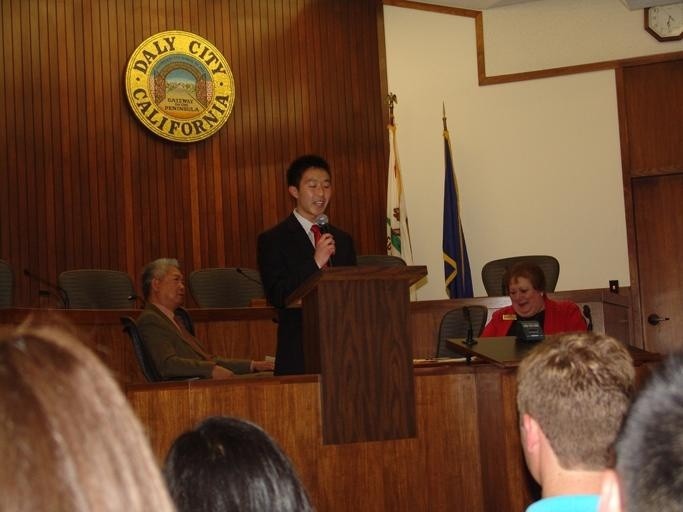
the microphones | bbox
[235,265,262,287]
[22,267,71,308]
[461,305,474,341]
[127,293,145,306]
[315,213,337,266]
[583,304,593,332]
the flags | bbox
[381,124,425,304]
[439,129,474,300]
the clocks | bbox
[643,2,683,42]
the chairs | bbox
[119,305,202,382]
[190,266,266,307]
[438,304,489,358]
[0,261,13,305]
[58,269,136,310]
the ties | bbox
[310,225,329,272]
[173,315,212,362]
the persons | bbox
[597,346,682,511]
[132,257,278,382]
[514,328,637,511]
[474,260,590,341]
[163,411,316,511]
[254,151,358,377]
[1,306,182,512]
